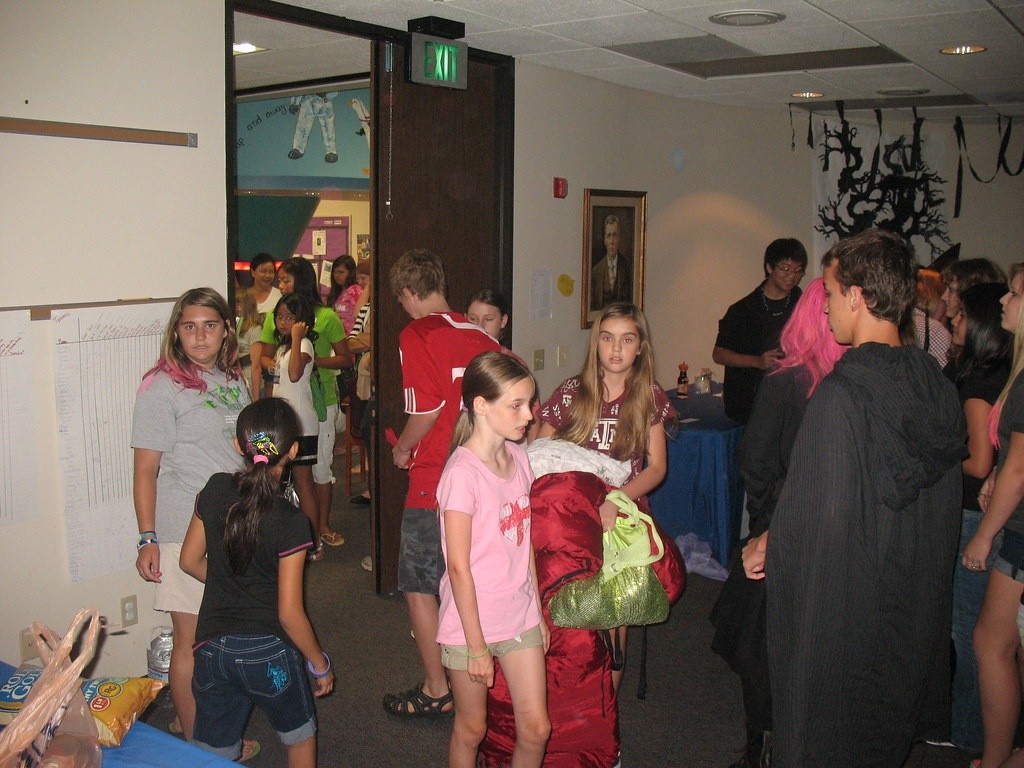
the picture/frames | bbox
[580,188,649,330]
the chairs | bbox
[341,395,368,498]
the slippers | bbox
[321,531,345,546]
[233,739,261,764]
[169,723,184,734]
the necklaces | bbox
[762,287,791,316]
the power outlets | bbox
[20,627,39,662]
[121,594,138,629]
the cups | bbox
[695,376,710,394]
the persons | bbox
[129,286,262,764]
[233,253,371,573]
[382,251,503,717]
[435,350,554,768]
[535,303,678,698]
[411,288,542,637]
[709,233,1023,767]
[178,396,336,768]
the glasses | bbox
[774,263,805,278]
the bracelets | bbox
[308,652,331,679]
[136,531,157,551]
[467,644,490,659]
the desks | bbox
[101,719,248,768]
[640,380,746,567]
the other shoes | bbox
[351,495,371,506]
[361,555,373,572]
[306,543,326,562]
[921,725,957,748]
[351,461,368,473]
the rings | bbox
[972,564,980,569]
[140,569,143,574]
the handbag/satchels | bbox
[547,490,669,631]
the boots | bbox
[724,723,773,768]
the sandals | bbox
[384,683,456,721]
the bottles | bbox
[676,362,688,399]
[147,627,174,682]
